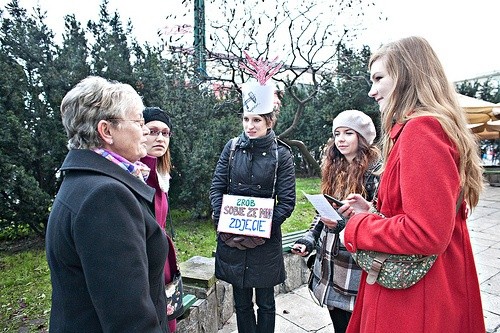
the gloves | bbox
[219,232,266,250]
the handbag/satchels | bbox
[348,207,439,289]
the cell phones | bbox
[324,194,344,207]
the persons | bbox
[141,107,181,333]
[333,36,486,333]
[291,110,383,333]
[209,81,296,333]
[45,76,169,333]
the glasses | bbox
[111,118,145,128]
[149,129,171,138]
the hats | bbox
[143,107,172,129]
[237,52,282,116]
[332,109,377,146]
